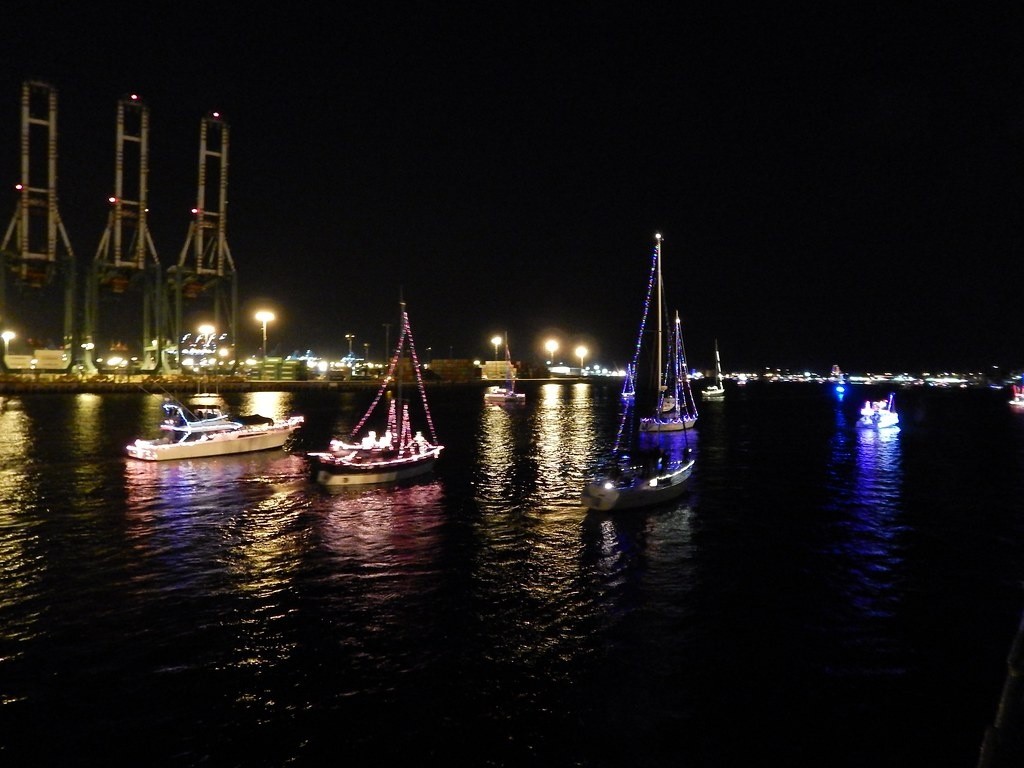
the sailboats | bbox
[311,301,444,495]
[485,233,728,509]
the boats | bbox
[1008,380,1024,417]
[852,393,902,431]
[126,380,309,462]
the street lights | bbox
[490,336,503,380]
[344,331,357,366]
[254,307,277,371]
[198,320,217,374]
[80,339,96,379]
[544,336,561,367]
[574,342,590,372]
